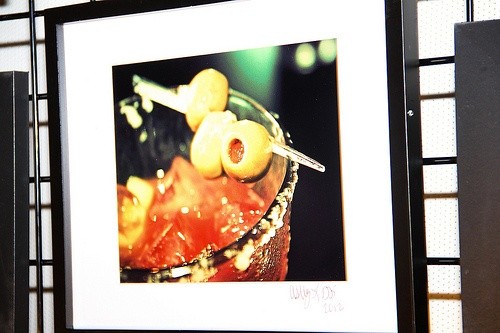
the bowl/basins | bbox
[114,87,298,283]
